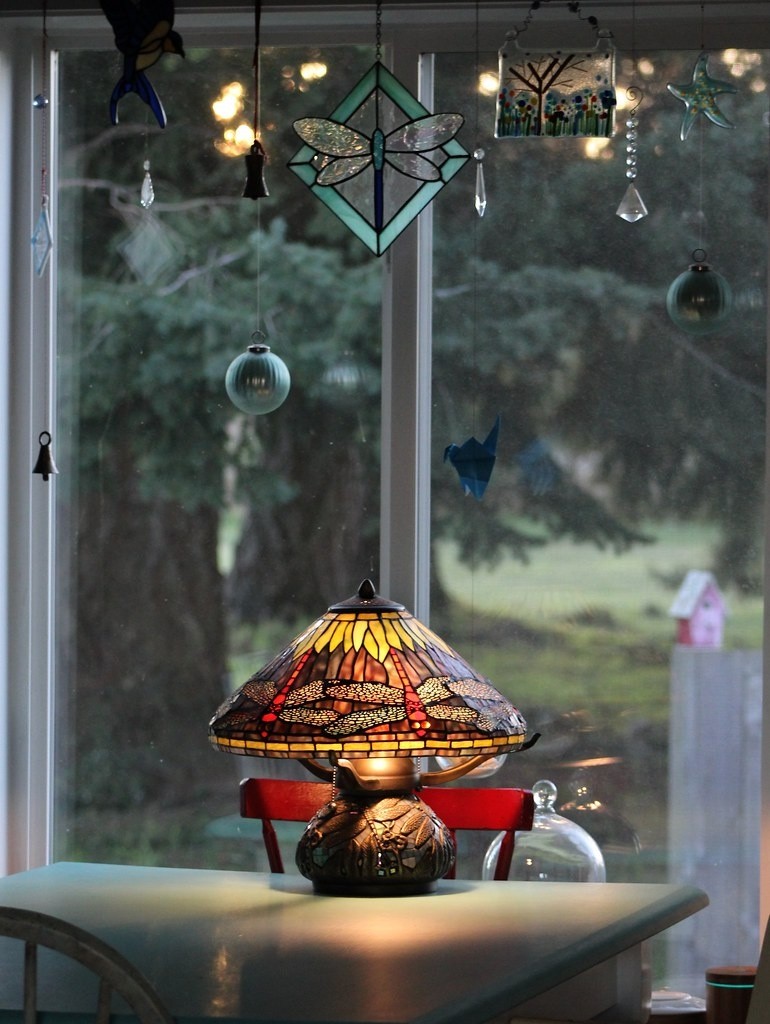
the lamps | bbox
[209,580,539,897]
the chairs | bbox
[237,777,533,882]
[0,907,173,1024]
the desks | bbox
[0,862,711,1024]
